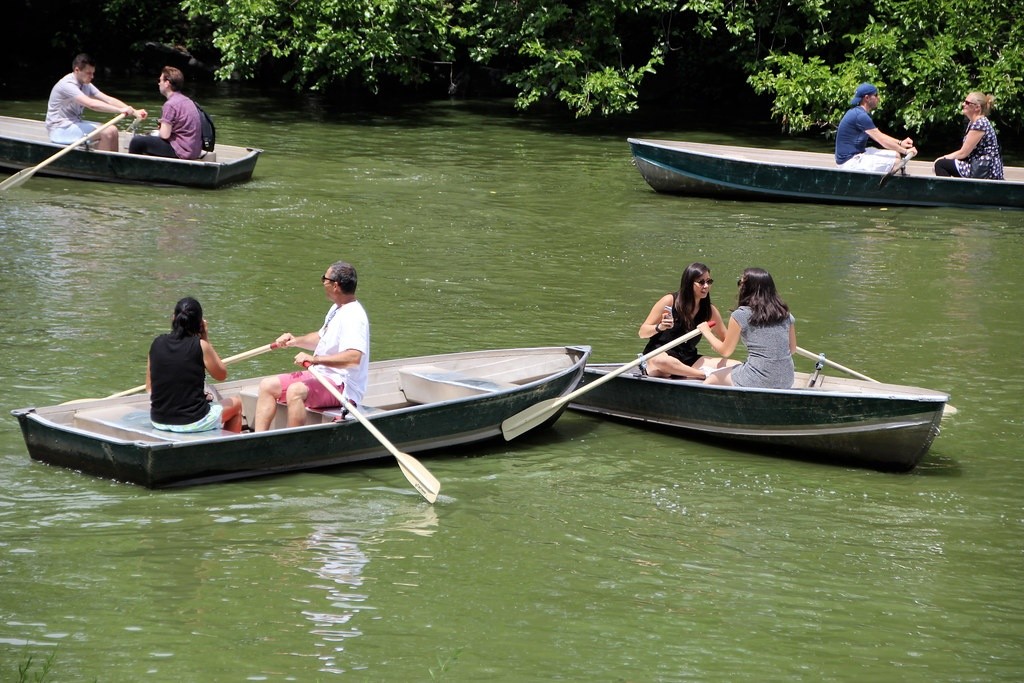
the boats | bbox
[627,136,1024,211]
[10,344,593,489]
[0,116,265,188]
[566,362,952,473]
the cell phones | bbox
[664,306,672,327]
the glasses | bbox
[696,278,714,285]
[966,101,976,105]
[321,275,335,283]
[737,280,744,287]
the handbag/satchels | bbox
[194,102,216,160]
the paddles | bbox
[303,361,441,504]
[60,342,276,404]
[797,346,954,408]
[879,151,914,188]
[0,112,127,190]
[129,112,146,131]
[502,319,717,442]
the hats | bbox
[851,84,877,105]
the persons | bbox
[696,267,797,389]
[255,261,370,433]
[129,65,203,160]
[45,54,148,153]
[835,83,918,176]
[146,297,242,434]
[638,263,742,381]
[933,92,1004,181]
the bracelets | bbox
[655,322,663,333]
[898,140,902,145]
[313,354,319,366]
[944,155,946,159]
